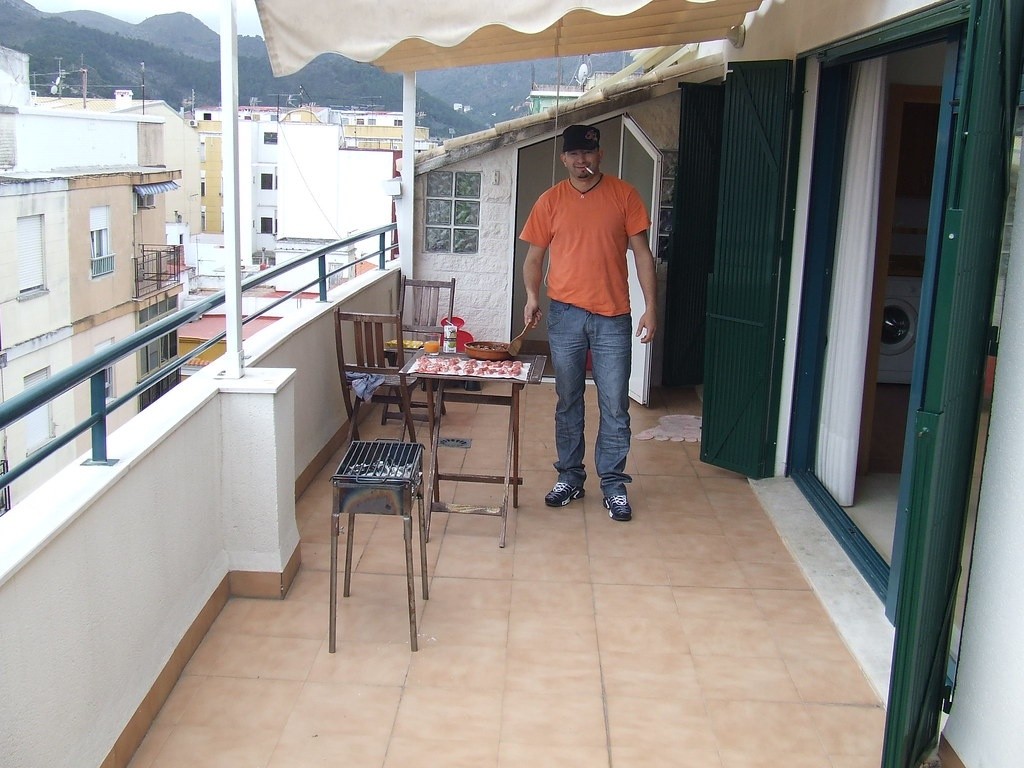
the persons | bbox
[519,125,657,521]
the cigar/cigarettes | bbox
[585,167,594,175]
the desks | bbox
[397,347,547,548]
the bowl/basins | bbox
[464,342,511,361]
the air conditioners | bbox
[188,119,198,128]
[138,193,156,207]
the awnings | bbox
[257,1,758,77]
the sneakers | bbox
[544,481,585,507]
[602,493,632,521]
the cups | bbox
[424,333,441,355]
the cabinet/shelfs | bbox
[897,100,940,202]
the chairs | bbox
[331,309,422,443]
[378,275,455,424]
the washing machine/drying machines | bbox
[875,274,922,385]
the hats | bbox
[561,124,601,152]
